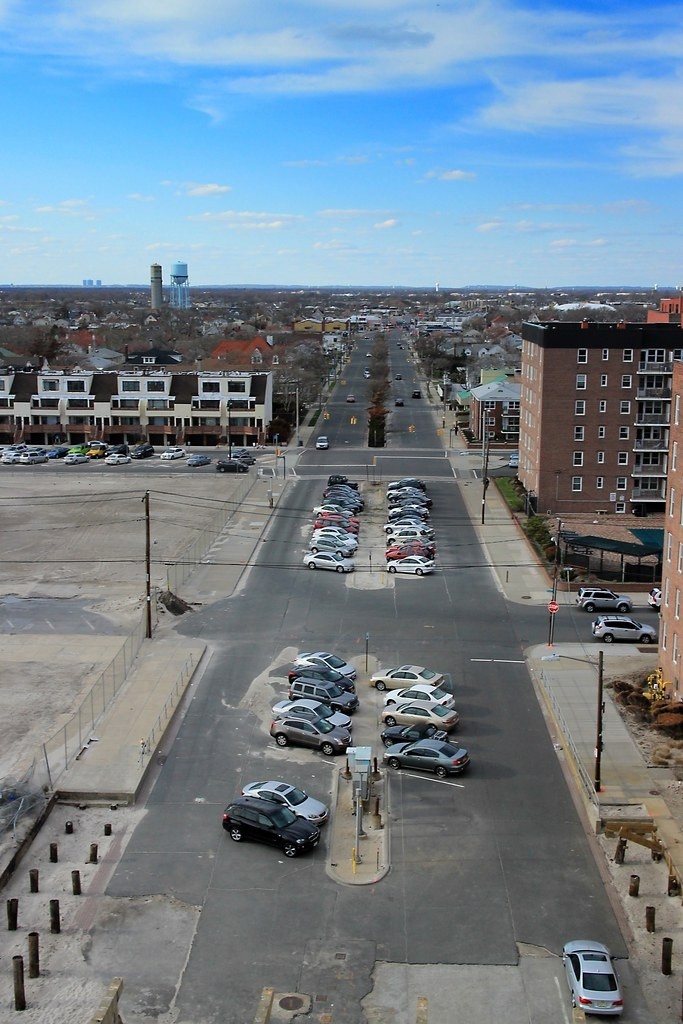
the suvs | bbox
[576,587,661,643]
[412,390,421,399]
[223,796,321,858]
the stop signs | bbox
[548,601,559,613]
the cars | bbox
[0,442,255,473]
[364,367,371,378]
[303,475,364,575]
[242,781,331,826]
[270,652,361,756]
[347,395,355,402]
[371,664,470,778]
[316,437,329,450]
[395,398,404,406]
[562,940,624,1015]
[509,450,519,467]
[382,477,435,576]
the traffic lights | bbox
[351,417,356,424]
[437,429,443,436]
[324,412,329,420]
[409,424,415,433]
